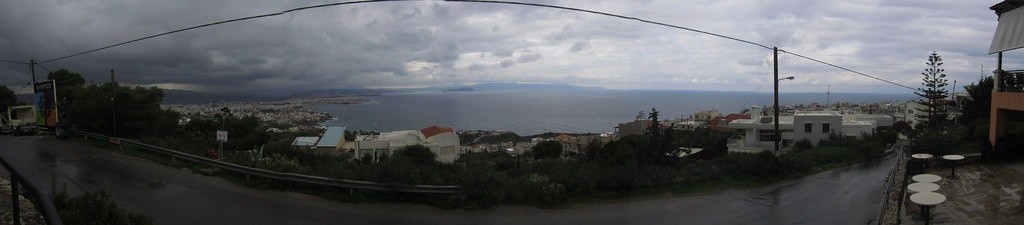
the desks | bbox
[912,173,942,183]
[907,182,940,221]
[909,192,946,225]
[912,154,934,173]
[943,154,965,179]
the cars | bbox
[0,115,9,136]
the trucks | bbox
[9,104,37,135]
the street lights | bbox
[773,75,795,153]
